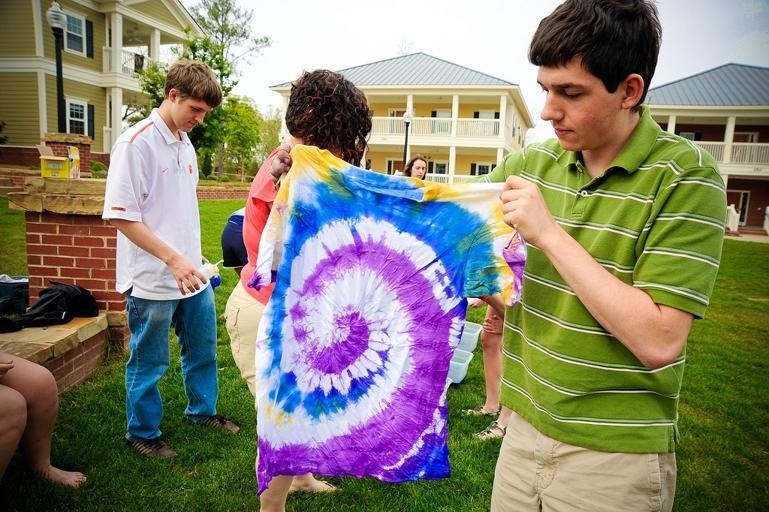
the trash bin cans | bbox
[0,276,28,333]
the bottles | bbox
[181,258,224,294]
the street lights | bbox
[44,1,67,133]
[401,110,411,172]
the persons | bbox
[268,0,730,512]
[225,66,377,512]
[398,155,429,182]
[99,58,243,465]
[459,291,513,444]
[220,204,256,278]
[1,350,91,493]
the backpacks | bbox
[22,278,99,327]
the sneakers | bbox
[187,414,241,435]
[459,406,501,418]
[472,419,507,443]
[126,436,177,462]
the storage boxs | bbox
[447,321,482,383]
[35,143,81,180]
